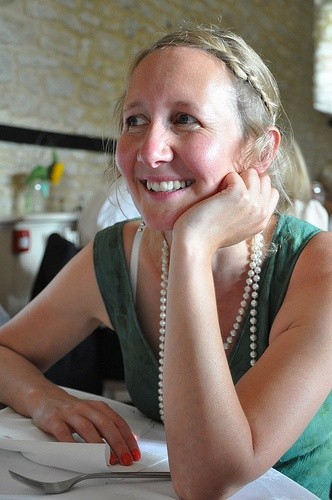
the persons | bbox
[0,22,332,500]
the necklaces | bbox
[154,231,264,427]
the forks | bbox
[9,470,172,494]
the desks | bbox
[0,385,321,500]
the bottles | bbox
[25,161,67,212]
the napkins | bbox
[0,387,168,475]
